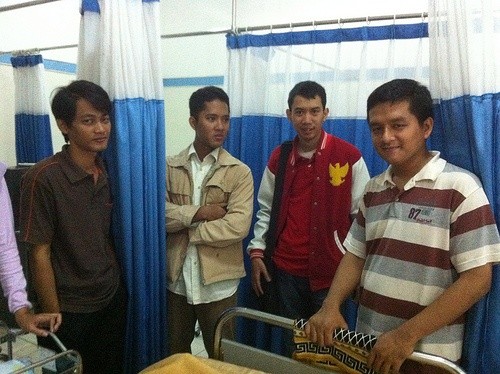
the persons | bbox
[18,80,129,374]
[164,85,255,360]
[0,160,62,338]
[247,80,370,357]
[307,78,500,374]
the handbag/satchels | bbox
[244,259,279,325]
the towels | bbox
[293,317,392,374]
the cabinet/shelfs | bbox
[0,321,81,374]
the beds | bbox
[134,308,467,374]
